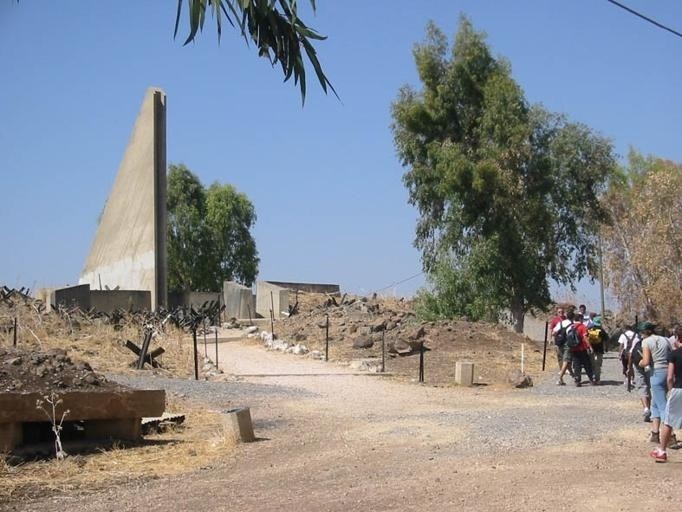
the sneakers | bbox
[556,369,601,387]
[648,447,667,462]
[647,430,660,444]
[642,409,651,421]
[667,435,677,448]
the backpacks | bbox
[555,321,603,347]
[623,332,652,369]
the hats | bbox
[637,322,656,331]
[593,317,602,326]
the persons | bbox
[617,320,682,463]
[549,305,610,386]
